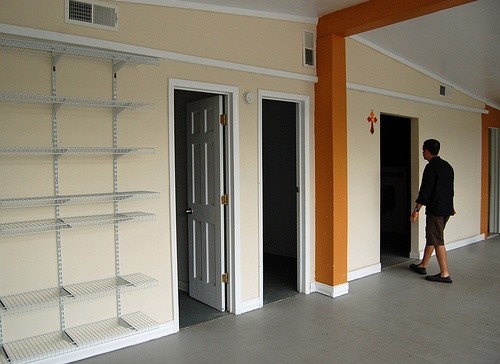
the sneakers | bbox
[424,273,453,283]
[410,263,426,274]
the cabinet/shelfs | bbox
[0,39,161,364]
